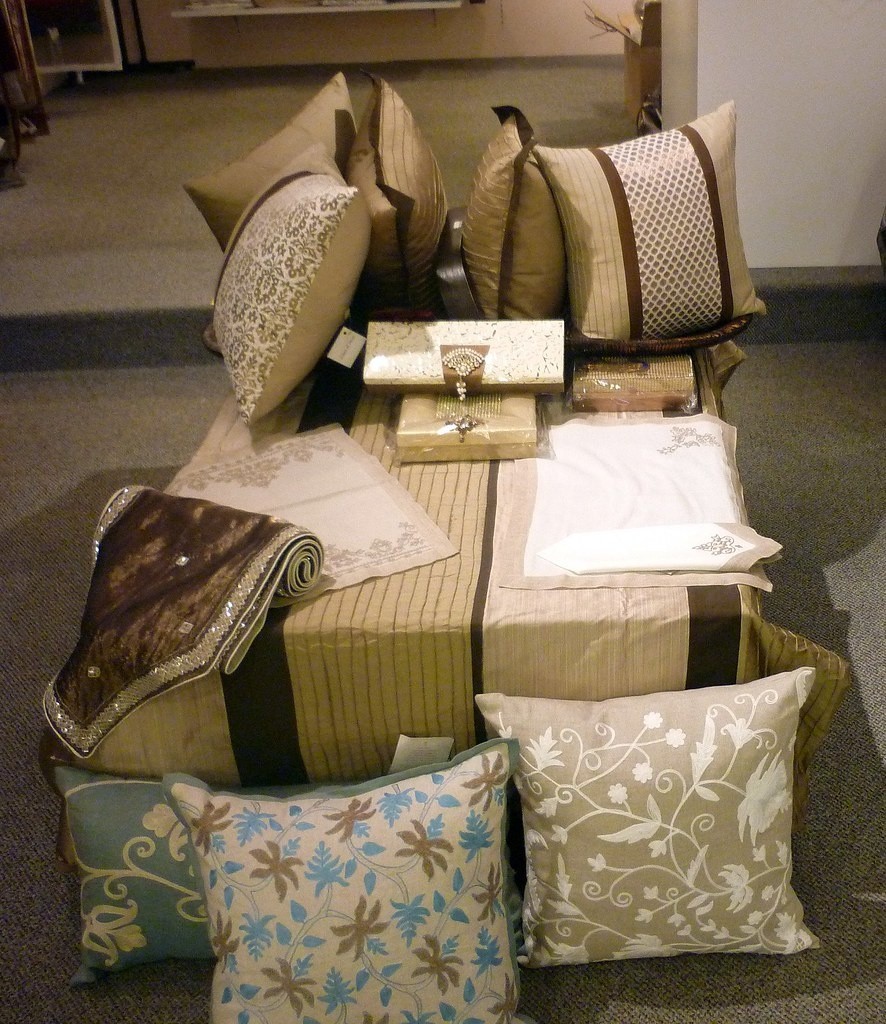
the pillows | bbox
[348,71,448,321]
[461,103,569,319]
[48,757,359,985]
[477,666,823,966]
[184,70,360,248]
[533,100,769,348]
[210,142,376,424]
[161,737,537,1024]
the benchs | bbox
[69,348,762,779]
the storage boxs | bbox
[362,317,568,393]
[572,351,698,413]
[397,392,538,462]
[326,327,368,368]
